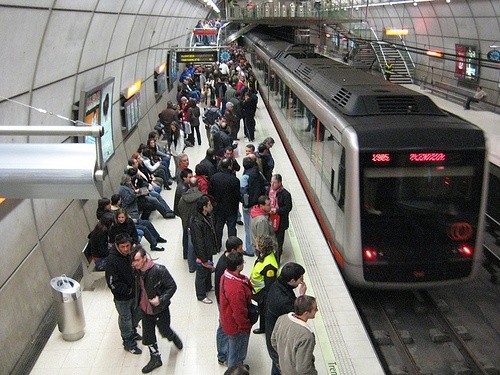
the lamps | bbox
[122,80,141,99]
[0,124,105,200]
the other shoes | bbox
[151,246,164,251]
[242,365,249,370]
[218,360,224,365]
[211,286,215,291]
[173,333,183,350]
[142,354,163,373]
[237,221,243,225]
[157,237,167,243]
[164,213,175,219]
[253,329,266,334]
[243,251,255,257]
[202,297,213,304]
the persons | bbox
[463,87,486,110]
[87,19,320,375]
[384,59,392,81]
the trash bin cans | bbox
[50,277,85,342]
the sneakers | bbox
[125,346,142,355]
[135,333,141,340]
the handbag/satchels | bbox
[95,260,106,271]
[270,213,280,231]
[140,187,149,196]
[191,117,199,126]
[248,303,259,324]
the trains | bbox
[241,30,491,293]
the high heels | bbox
[164,183,172,190]
